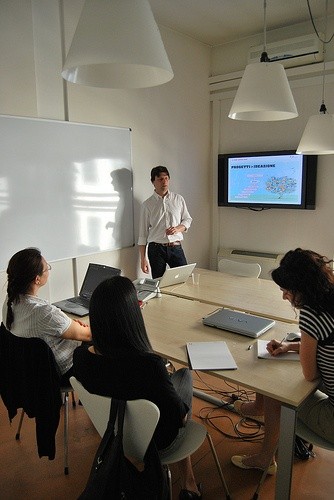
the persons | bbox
[137,165,192,280]
[72,275,204,500]
[231,248,334,475]
[2,247,93,405]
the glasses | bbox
[40,262,52,275]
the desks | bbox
[64,294,320,500]
[161,267,299,325]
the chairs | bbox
[0,322,77,475]
[252,416,334,500]
[218,259,262,279]
[69,376,228,500]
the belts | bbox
[152,241,182,247]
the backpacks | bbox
[77,397,169,500]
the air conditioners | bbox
[248,33,324,68]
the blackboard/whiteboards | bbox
[0,114,136,273]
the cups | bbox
[192,272,200,284]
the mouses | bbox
[286,332,302,342]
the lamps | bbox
[296,0,334,155]
[228,0,299,121]
[61,0,174,89]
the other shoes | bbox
[232,455,278,475]
[234,400,265,423]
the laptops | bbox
[203,309,275,338]
[51,263,121,316]
[139,262,196,288]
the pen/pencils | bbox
[270,338,285,357]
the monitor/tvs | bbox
[217,150,318,210]
